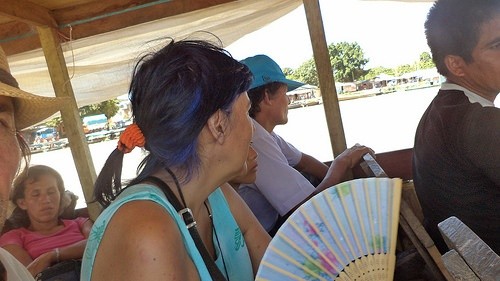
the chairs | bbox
[439,213,500,281]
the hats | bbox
[239,54,305,91]
[0,46,70,131]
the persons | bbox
[228,54,376,237]
[0,46,94,281]
[79,30,274,280]
[412,0,500,253]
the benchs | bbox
[359,150,454,281]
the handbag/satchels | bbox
[34,260,82,281]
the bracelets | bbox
[55,247,63,263]
